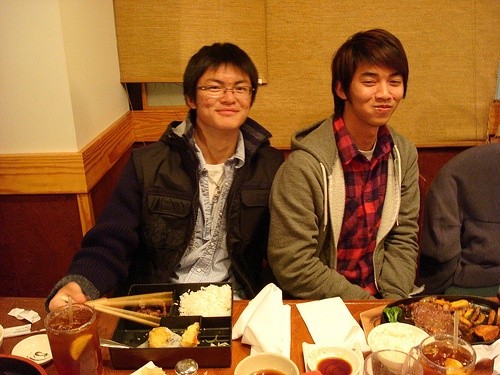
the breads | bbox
[148,322,200,348]
[473,325,499,342]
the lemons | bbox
[70,334,92,360]
[445,358,465,375]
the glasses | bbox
[197,84,255,98]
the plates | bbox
[11,334,53,365]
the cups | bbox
[418,335,476,375]
[364,350,424,375]
[43,304,105,375]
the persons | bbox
[45,43,285,313]
[417,140,500,297]
[267,27,421,300]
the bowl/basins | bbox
[367,322,435,373]
[382,295,500,346]
[233,353,299,375]
[307,344,364,375]
[0,324,3,349]
[0,353,47,375]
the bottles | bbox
[174,358,199,375]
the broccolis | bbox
[382,306,402,323]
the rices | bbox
[174,284,232,317]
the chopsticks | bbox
[87,291,173,307]
[84,301,160,327]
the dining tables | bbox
[0,281,500,375]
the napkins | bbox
[231,283,291,361]
[296,296,372,352]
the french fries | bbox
[434,298,500,330]
